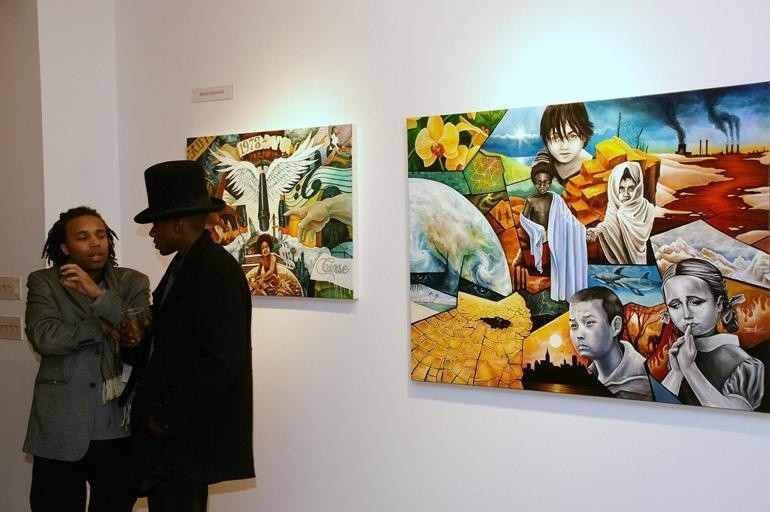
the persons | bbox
[107,157,258,511]
[566,285,655,402]
[512,159,597,268]
[21,204,155,511]
[283,192,352,243]
[585,159,663,274]
[327,127,342,157]
[247,233,279,296]
[641,256,765,412]
[530,102,597,197]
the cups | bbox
[120,308,147,343]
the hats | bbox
[134,160,226,223]
